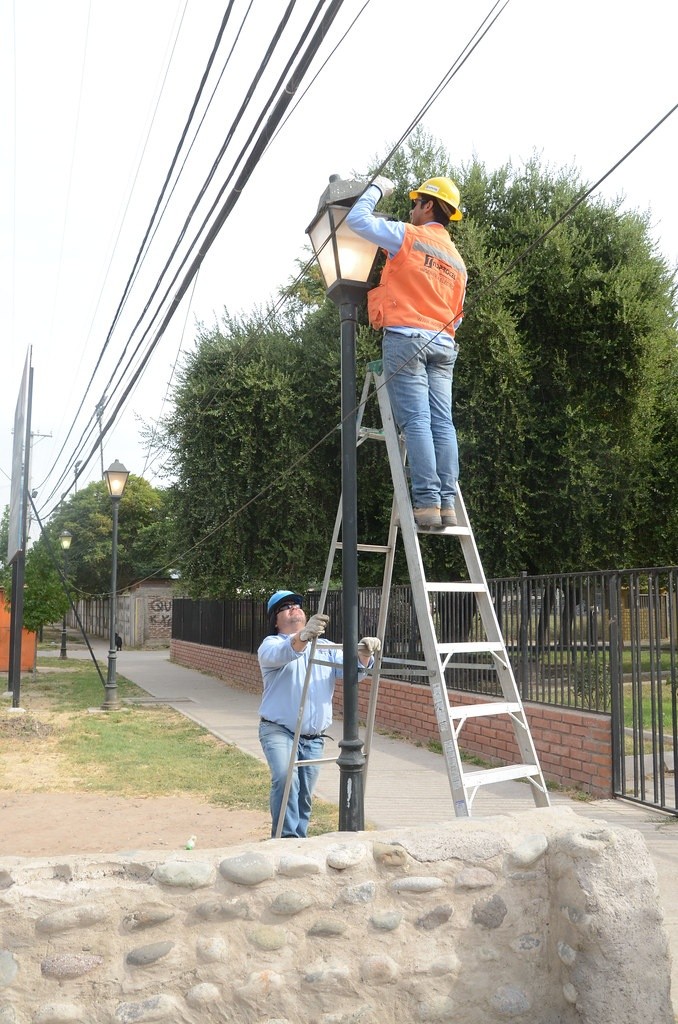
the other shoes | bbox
[393,507,442,527]
[440,507,457,526]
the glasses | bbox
[411,198,429,211]
[275,602,302,615]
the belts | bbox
[260,717,334,741]
[383,328,391,336]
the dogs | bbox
[115,632,122,651]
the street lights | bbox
[302,172,395,834]
[58,530,74,663]
[100,458,130,710]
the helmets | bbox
[409,177,462,220]
[267,590,299,621]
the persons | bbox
[256,589,382,838]
[345,175,465,527]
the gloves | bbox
[358,637,382,658]
[299,614,329,642]
[369,173,396,199]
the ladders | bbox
[269,358,552,839]
